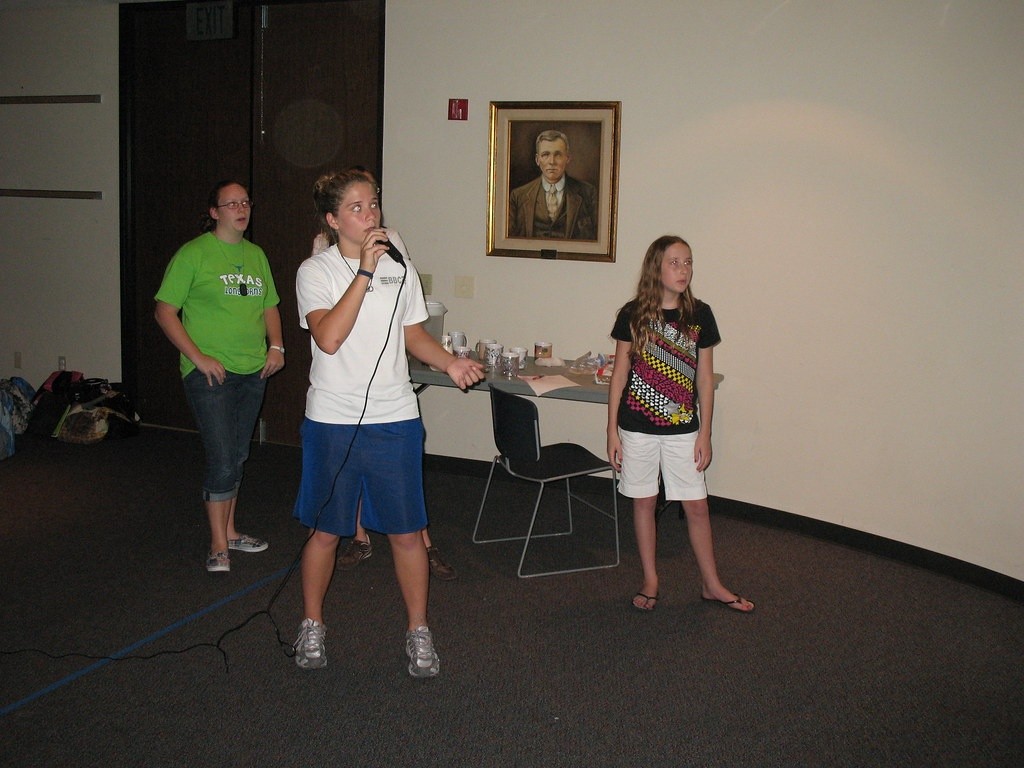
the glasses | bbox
[374,186,380,194]
[216,201,254,209]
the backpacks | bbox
[25,368,86,438]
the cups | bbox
[534,342,553,360]
[484,344,504,365]
[496,352,519,377]
[510,347,528,369]
[475,339,497,360]
[430,336,452,372]
[453,347,470,358]
[448,331,467,349]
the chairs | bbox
[473,383,620,578]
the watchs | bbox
[269,345,285,353]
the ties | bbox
[548,184,558,223]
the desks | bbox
[408,352,725,532]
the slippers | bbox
[631,591,658,613]
[702,589,756,614]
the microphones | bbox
[377,240,405,267]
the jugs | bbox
[420,301,448,343]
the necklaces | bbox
[216,238,247,296]
[337,246,373,292]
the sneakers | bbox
[293,619,329,670]
[405,625,440,680]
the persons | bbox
[153,178,286,572]
[292,165,486,678]
[606,235,755,614]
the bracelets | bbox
[357,269,373,279]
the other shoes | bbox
[337,534,374,572]
[427,546,456,582]
[207,550,230,572]
[227,535,268,553]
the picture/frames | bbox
[485,101,622,263]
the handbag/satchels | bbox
[57,402,130,445]
